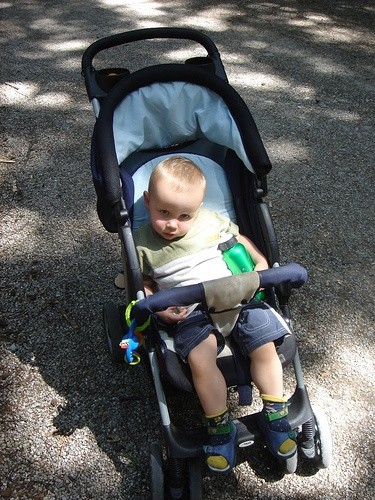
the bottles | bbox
[218,233,265,302]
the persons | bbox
[129,155,299,474]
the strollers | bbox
[81,26,333,500]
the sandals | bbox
[264,406,298,458]
[203,421,237,472]
